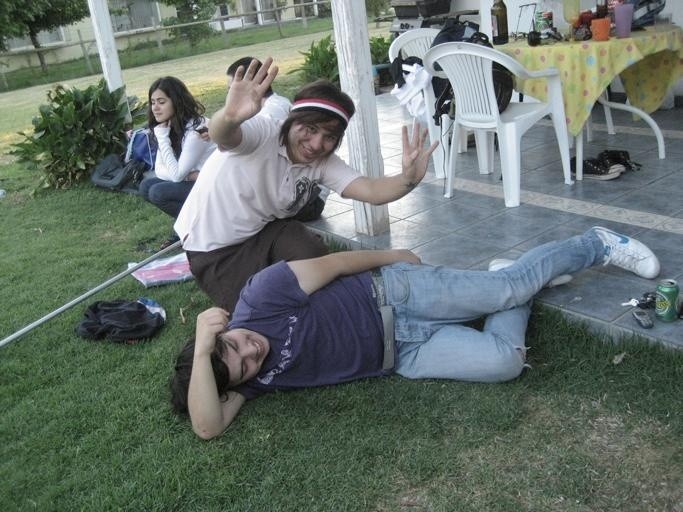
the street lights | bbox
[426,16,515,121]
[125,126,156,170]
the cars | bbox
[653,14,672,31]
[615,5,633,39]
[591,18,610,40]
[527,31,541,46]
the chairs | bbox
[487,259,572,288]
[568,150,628,181]
[590,226,661,279]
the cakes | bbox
[534,11,553,33]
[654,279,678,323]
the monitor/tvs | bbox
[126,249,194,289]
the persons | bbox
[170,226,660,441]
[177,55,438,323]
[222,56,294,123]
[138,76,218,220]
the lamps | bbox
[422,43,573,209]
[389,27,495,183]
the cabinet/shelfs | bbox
[490,1,508,46]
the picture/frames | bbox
[467,23,681,162]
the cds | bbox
[621,292,657,307]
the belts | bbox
[561,1,579,42]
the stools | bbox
[632,2,665,21]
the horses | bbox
[633,310,653,329]
